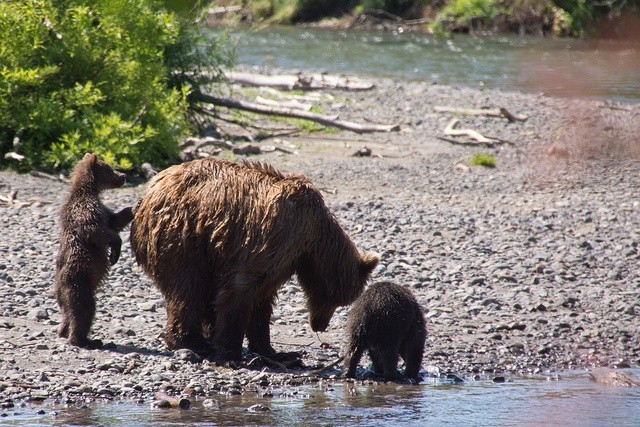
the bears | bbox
[129,157,380,361]
[344,282,427,381]
[56,152,134,346]
